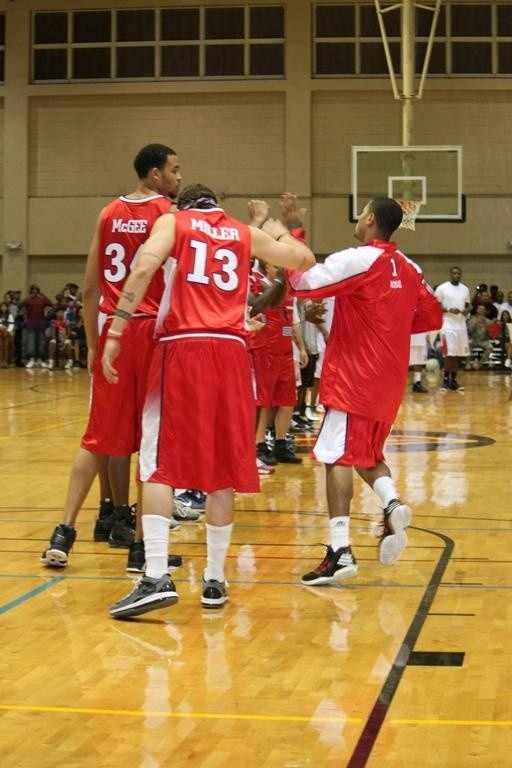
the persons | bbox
[246,199,271,230]
[274,192,441,593]
[94,450,139,547]
[99,182,318,616]
[40,144,183,575]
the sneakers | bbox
[376,498,414,567]
[24,357,86,371]
[39,524,77,568]
[412,381,428,395]
[168,489,211,530]
[92,505,136,546]
[126,538,182,571]
[255,402,326,476]
[300,543,360,586]
[107,572,179,619]
[441,381,465,394]
[197,577,230,609]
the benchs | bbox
[461,340,511,366]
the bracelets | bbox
[116,308,132,321]
[276,231,289,242]
[106,329,124,339]
